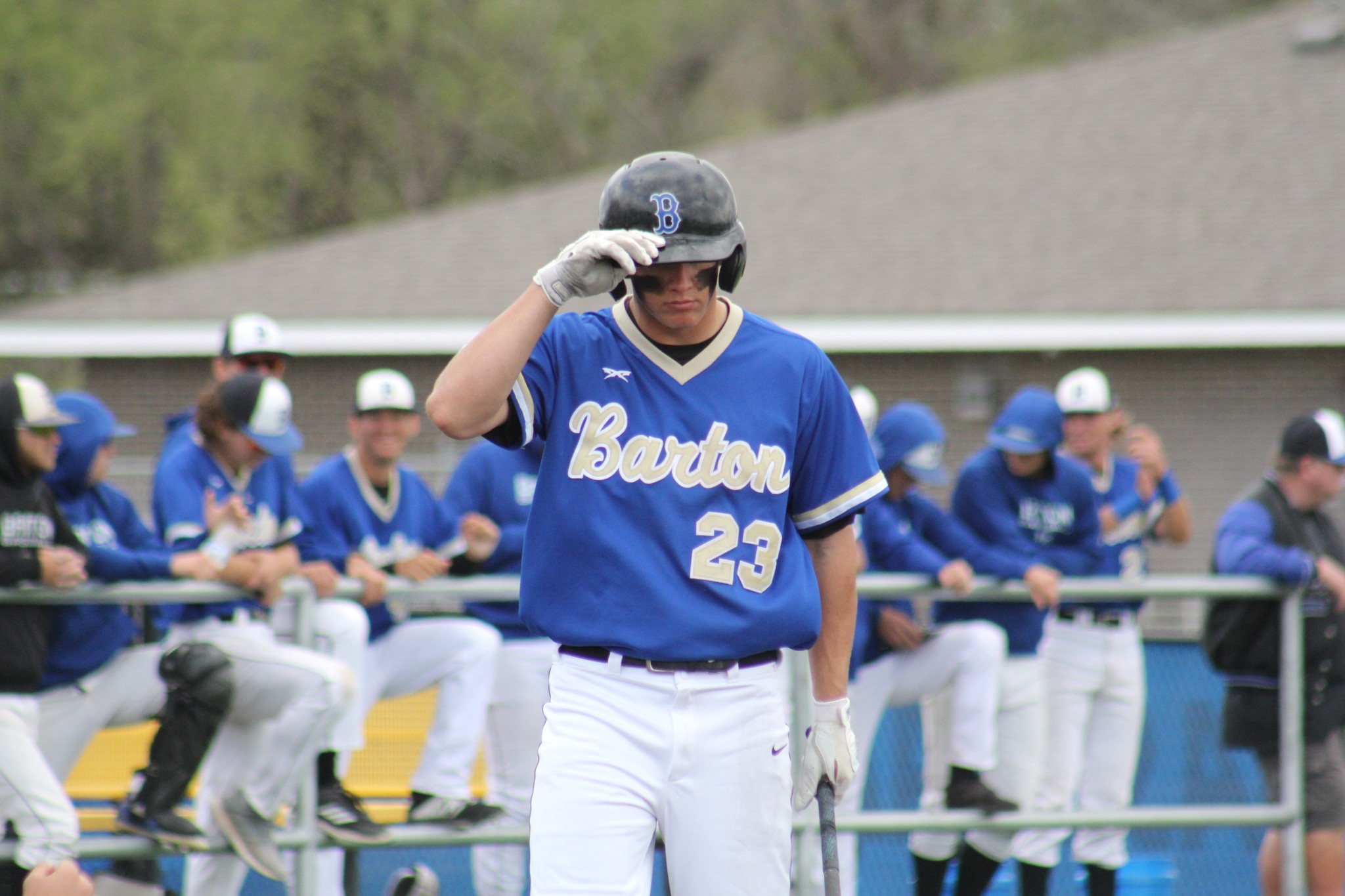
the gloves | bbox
[534,230,666,306]
[791,695,857,811]
[198,503,307,569]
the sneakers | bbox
[408,790,509,827]
[113,796,210,851]
[319,785,393,846]
[213,792,289,880]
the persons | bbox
[1009,364,1193,896]
[0,311,1193,896]
[150,371,358,896]
[0,370,93,896]
[907,389,1105,896]
[437,440,562,896]
[1201,404,1344,896]
[40,392,252,841]
[423,150,892,896]
[796,402,1064,896]
[298,367,505,849]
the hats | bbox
[352,370,418,417]
[1059,367,1111,418]
[0,372,81,428]
[216,314,294,360]
[51,390,138,440]
[984,384,1063,454]
[219,371,307,457]
[1276,407,1343,468]
[871,400,949,485]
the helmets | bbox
[596,152,748,296]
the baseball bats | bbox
[807,724,842,896]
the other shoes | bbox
[946,782,1018,812]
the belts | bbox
[559,645,778,672]
